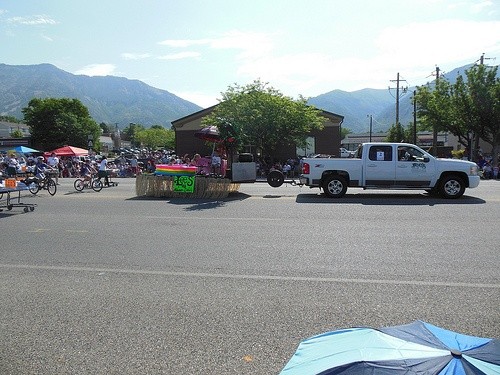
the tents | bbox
[43,145,88,157]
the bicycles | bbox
[28,171,57,196]
[73,172,103,192]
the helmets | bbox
[37,156,44,160]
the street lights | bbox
[367,114,373,142]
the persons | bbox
[463,146,500,180]
[0,144,301,185]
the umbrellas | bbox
[278,321,500,375]
[196,125,237,153]
[1,146,39,153]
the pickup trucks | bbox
[300,142,482,200]
